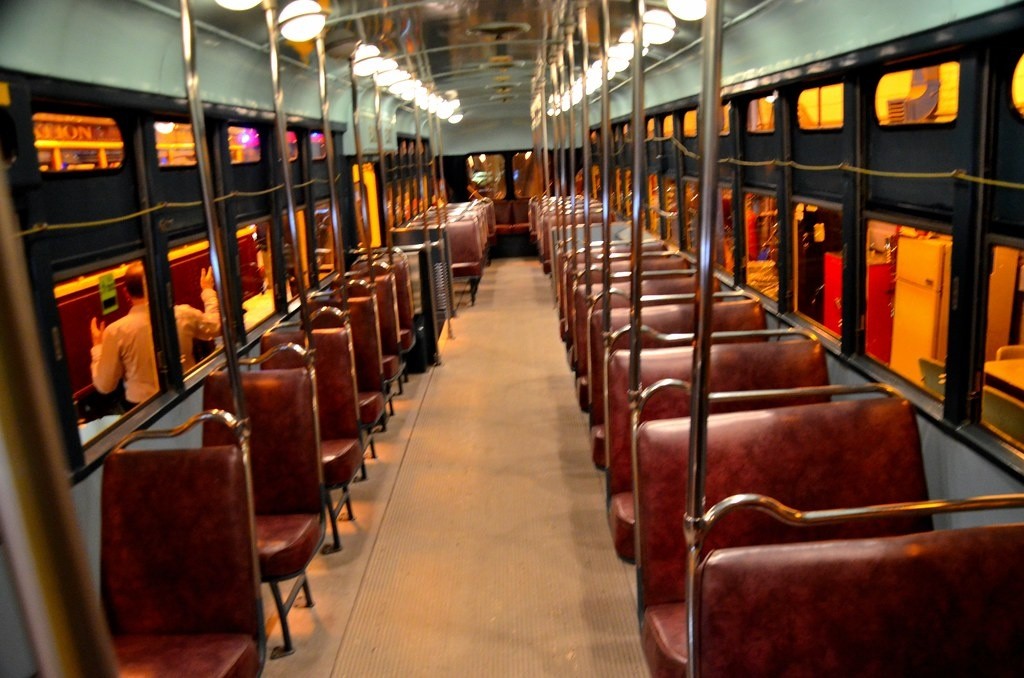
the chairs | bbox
[99,247,416,678]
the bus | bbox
[32,112,334,424]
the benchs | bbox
[389,195,1024,678]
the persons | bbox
[90,263,222,416]
